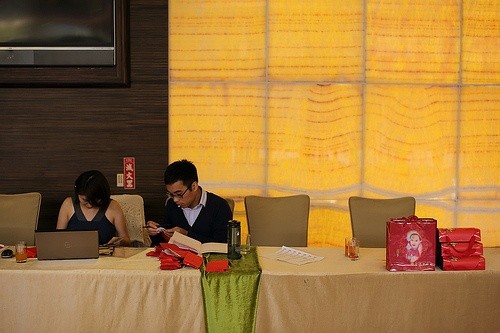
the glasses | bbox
[166,184,192,200]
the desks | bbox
[0,247,500,333]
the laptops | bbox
[34,229,99,260]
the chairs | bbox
[349,196,415,248]
[244,195,311,247]
[109,194,152,247]
[0,192,42,246]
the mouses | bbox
[1,249,13,258]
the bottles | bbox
[227,220,242,259]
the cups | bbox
[240,233,250,255]
[26,246,37,257]
[345,237,356,258]
[348,240,360,261]
[15,241,27,262]
[7,246,15,256]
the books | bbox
[168,231,228,256]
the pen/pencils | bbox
[142,225,167,228]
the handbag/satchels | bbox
[439,242,483,259]
[385,215,437,272]
[437,228,481,253]
[440,257,485,270]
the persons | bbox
[56,169,130,247]
[146,159,232,247]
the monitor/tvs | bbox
[0,0,117,67]
